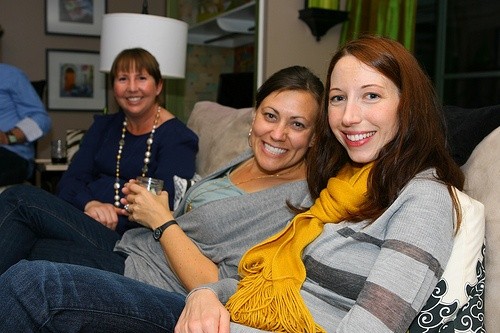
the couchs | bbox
[186,101,500,333]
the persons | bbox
[0,25,52,188]
[0,35,465,333]
[0,48,199,276]
[0,66,325,296]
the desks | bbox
[35,159,69,190]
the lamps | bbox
[299,0,352,42]
[98,13,188,80]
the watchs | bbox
[6,131,16,146]
[153,220,177,241]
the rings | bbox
[124,204,129,212]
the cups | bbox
[136,176,164,194]
[49,138,68,164]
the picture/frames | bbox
[45,48,109,111]
[44,0,108,39]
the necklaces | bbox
[186,160,304,214]
[114,106,160,207]
